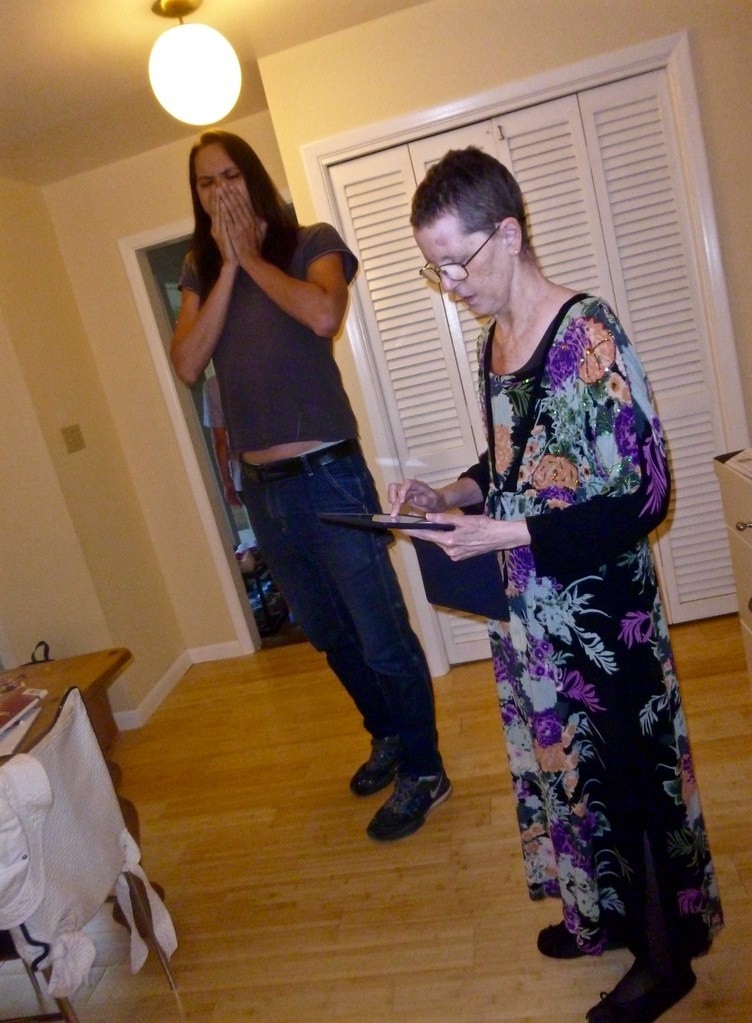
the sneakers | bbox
[366,771,452,843]
[349,736,400,795]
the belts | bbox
[240,440,356,484]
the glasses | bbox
[419,224,502,284]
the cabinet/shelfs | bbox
[712,448,752,674]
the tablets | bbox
[317,511,455,533]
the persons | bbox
[385,145,730,1023]
[168,129,455,844]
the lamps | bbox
[148,0,243,126]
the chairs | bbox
[0,685,179,1023]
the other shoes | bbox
[536,920,586,958]
[587,959,697,1023]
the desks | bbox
[0,647,166,931]
